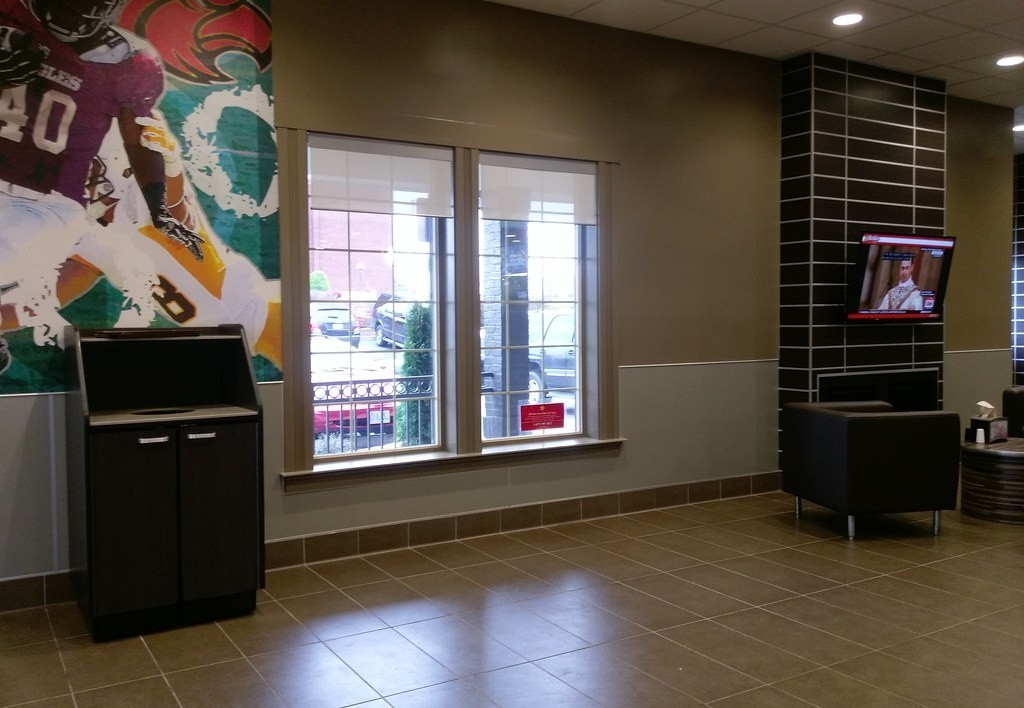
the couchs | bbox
[777,401,959,541]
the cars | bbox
[311,308,364,348]
[528,312,579,402]
[310,325,400,440]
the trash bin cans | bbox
[61,320,270,645]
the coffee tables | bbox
[959,438,1024,526]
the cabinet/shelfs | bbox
[64,326,266,643]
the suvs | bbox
[371,292,424,349]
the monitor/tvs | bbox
[842,230,956,323]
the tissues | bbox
[970,400,1009,444]
